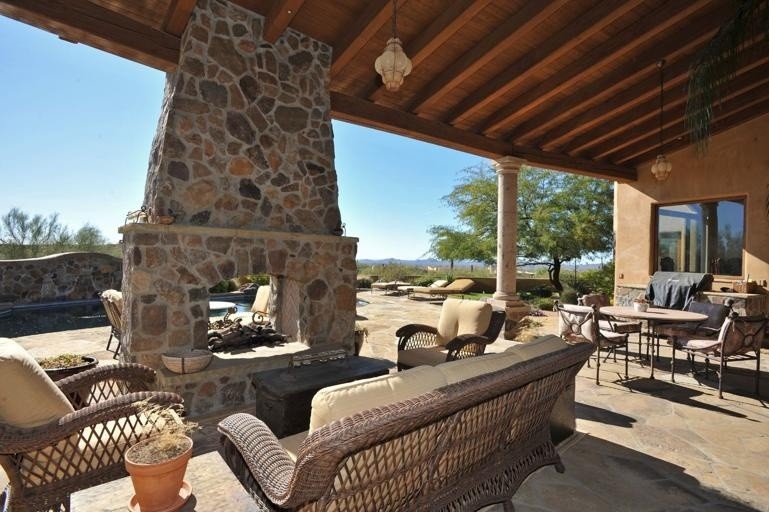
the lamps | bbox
[649,56,675,182]
[374,0,413,94]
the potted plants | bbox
[121,433,194,512]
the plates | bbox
[127,481,194,512]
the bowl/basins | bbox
[720,287,729,292]
[634,303,649,312]
[160,348,213,374]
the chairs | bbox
[0,332,187,512]
[369,273,477,303]
[393,296,507,374]
[551,287,768,400]
[97,288,123,359]
[251,283,272,325]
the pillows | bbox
[305,365,447,437]
[434,298,493,354]
[434,351,524,385]
[504,334,568,362]
[0,336,82,462]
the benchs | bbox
[216,332,596,511]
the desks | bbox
[209,301,237,326]
[67,450,261,512]
